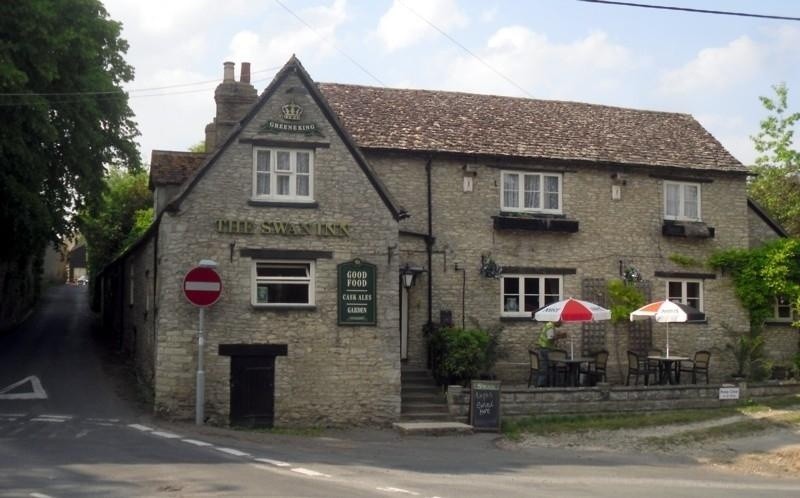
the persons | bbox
[540,319,567,357]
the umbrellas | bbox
[534,297,612,360]
[629,299,705,357]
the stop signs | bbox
[182,265,225,307]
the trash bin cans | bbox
[470,380,502,432]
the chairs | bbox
[525,344,713,390]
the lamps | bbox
[400,261,415,289]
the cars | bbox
[75,275,89,285]
